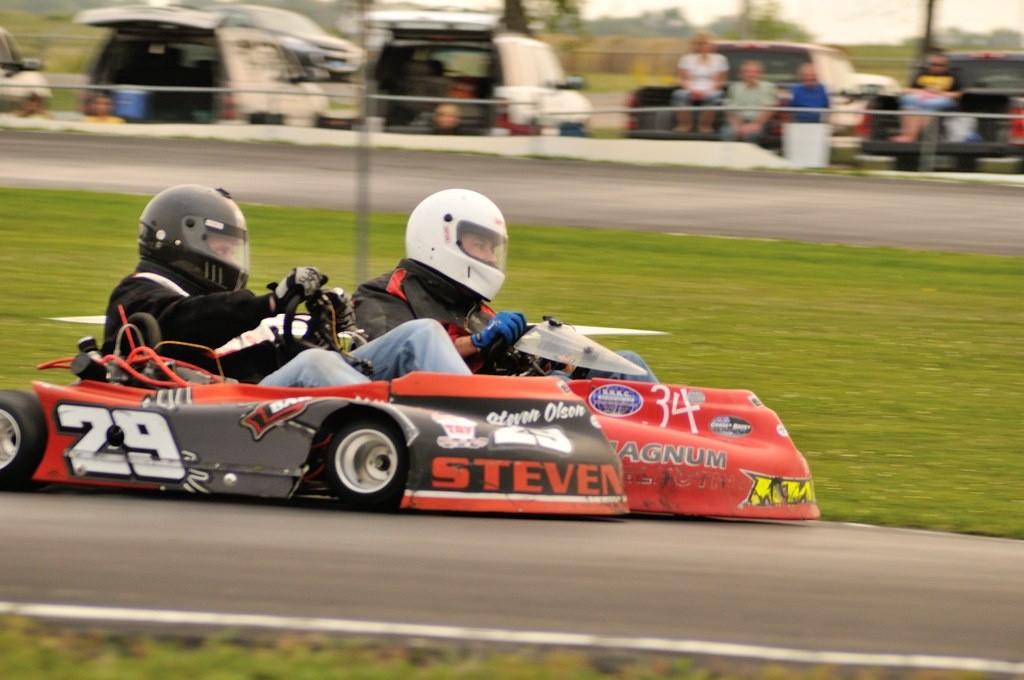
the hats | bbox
[498,0,530,25]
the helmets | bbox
[138,185,248,293]
[404,189,507,302]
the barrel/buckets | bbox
[111,91,148,123]
[781,124,831,167]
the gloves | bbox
[306,288,357,331]
[266,267,329,312]
[471,311,527,351]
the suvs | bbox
[73,8,329,129]
[356,12,592,135]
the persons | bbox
[101,183,473,389]
[720,61,779,144]
[350,187,661,383]
[788,64,828,123]
[668,30,729,133]
[76,92,125,124]
[16,95,57,120]
[432,101,465,135]
[890,54,962,141]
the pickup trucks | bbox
[625,40,869,149]
[861,52,1024,176]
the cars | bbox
[0,28,54,113]
[173,4,368,86]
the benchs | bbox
[637,86,770,131]
[861,140,1024,172]
[870,93,1010,169]
[621,131,781,147]
